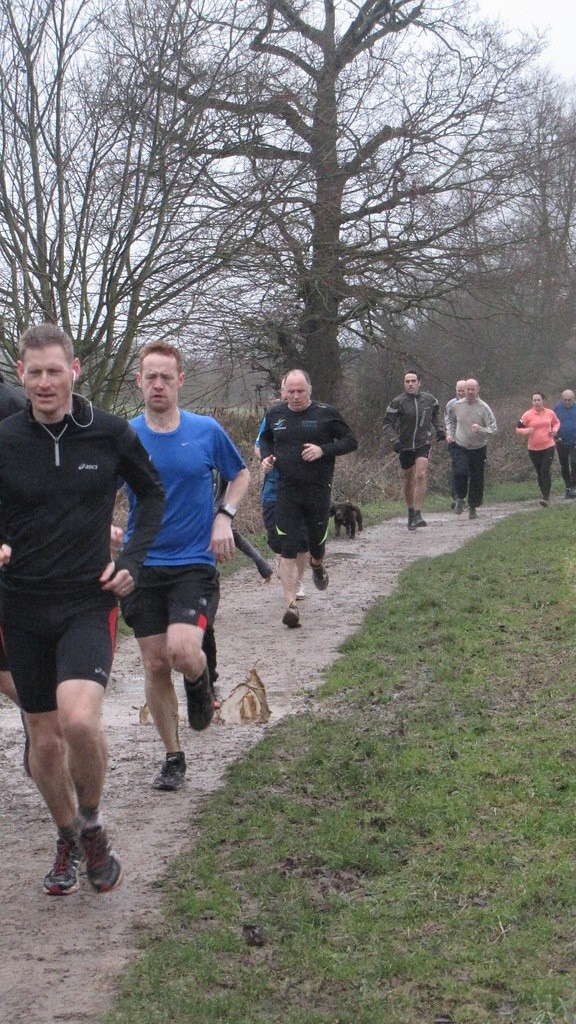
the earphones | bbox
[21,373,24,384]
[72,368,76,380]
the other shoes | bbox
[407,509,427,530]
[275,554,280,581]
[468,507,477,519]
[564,487,576,499]
[295,580,306,600]
[539,496,550,508]
[450,496,464,514]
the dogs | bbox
[328,500,363,539]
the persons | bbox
[382,370,446,530]
[0,323,250,896]
[254,369,358,628]
[552,389,576,499]
[515,393,561,508]
[443,379,496,519]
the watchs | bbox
[216,504,238,518]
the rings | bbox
[123,588,127,594]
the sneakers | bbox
[310,556,329,590]
[184,665,214,730]
[78,826,123,892]
[282,602,302,628]
[41,832,85,894]
[154,751,187,790]
